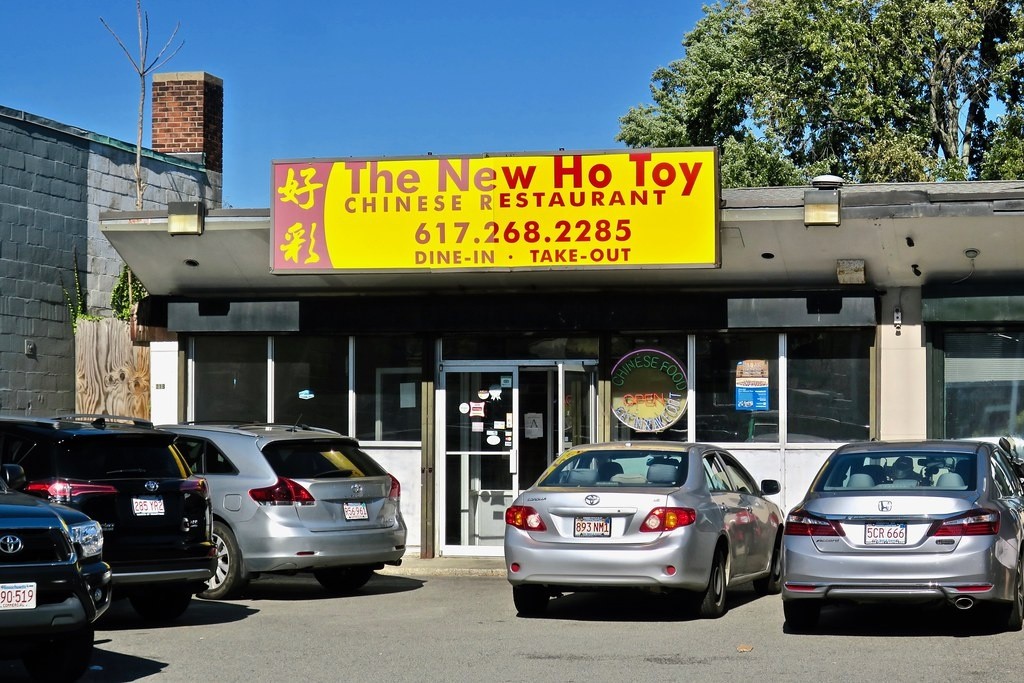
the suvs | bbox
[0,409,218,626]
[154,421,408,600]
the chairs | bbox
[846,459,974,489]
[566,464,679,486]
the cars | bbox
[502,437,785,623]
[1,464,111,683]
[778,437,1023,637]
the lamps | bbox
[166,201,206,236]
[802,175,844,227]
